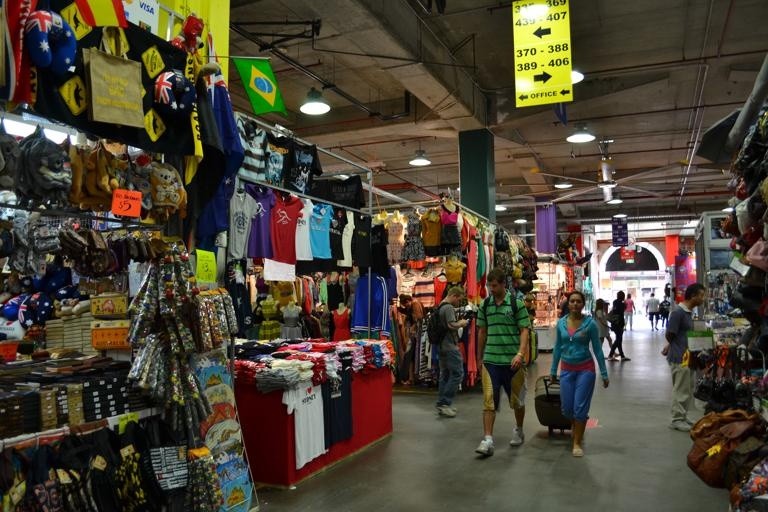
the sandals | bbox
[402,380,416,386]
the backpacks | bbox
[427,302,449,345]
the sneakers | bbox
[668,421,692,432]
[686,417,697,426]
[509,426,525,447]
[474,441,495,456]
[433,406,458,418]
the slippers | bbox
[572,448,584,458]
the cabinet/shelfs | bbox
[233,364,393,488]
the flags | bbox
[197,19,246,251]
[230,55,289,117]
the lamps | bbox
[565,124,595,145]
[612,209,627,219]
[299,89,332,117]
[554,178,573,190]
[606,194,624,205]
[408,150,431,167]
[513,215,527,224]
[570,67,584,85]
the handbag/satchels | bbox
[715,347,732,412]
[732,347,755,411]
[608,300,622,327]
[693,346,724,402]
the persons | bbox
[399,293,424,386]
[719,217,732,239]
[645,292,660,331]
[560,292,569,319]
[426,287,470,418]
[608,291,631,362]
[661,282,706,433]
[594,298,620,360]
[624,293,636,331]
[474,268,532,456]
[660,295,671,328]
[549,291,610,457]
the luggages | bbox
[534,377,572,435]
